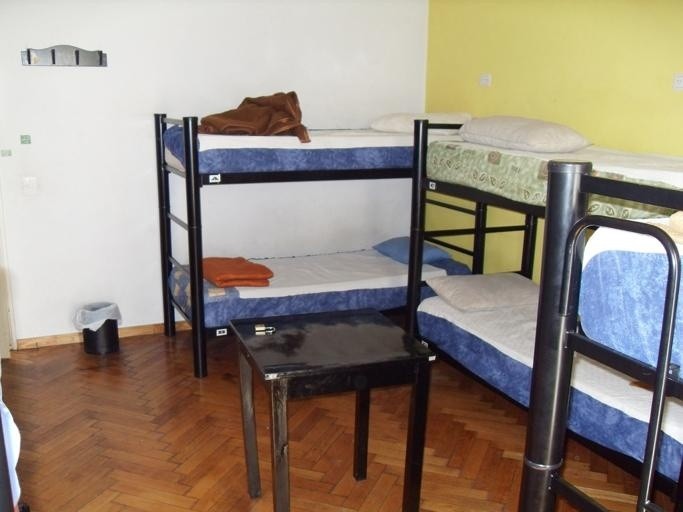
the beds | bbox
[155,114,487,379]
[409,117,682,510]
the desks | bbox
[227,307,437,511]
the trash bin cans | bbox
[77,303,121,356]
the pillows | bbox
[425,272,540,311]
[459,118,596,153]
[371,236,452,264]
